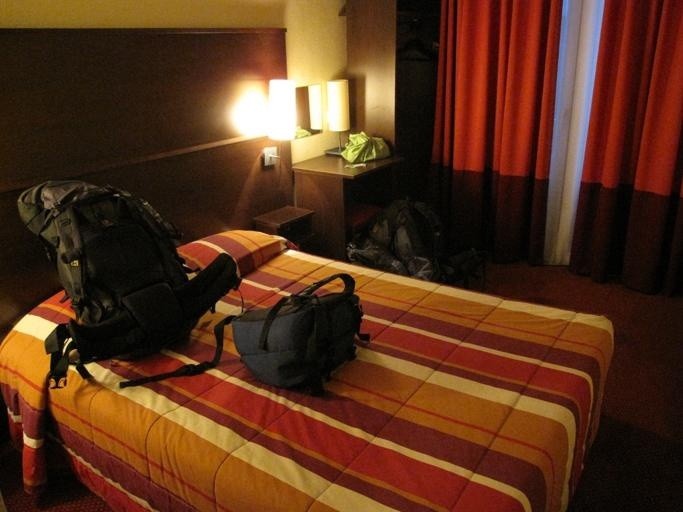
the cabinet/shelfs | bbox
[339,0,441,203]
[291,154,408,260]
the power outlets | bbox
[263,147,279,167]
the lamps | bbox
[325,79,351,156]
[266,78,294,160]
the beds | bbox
[0,225,615,512]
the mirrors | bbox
[296,85,323,139]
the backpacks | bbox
[230,272,369,392]
[16,180,241,389]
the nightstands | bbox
[252,204,315,240]
